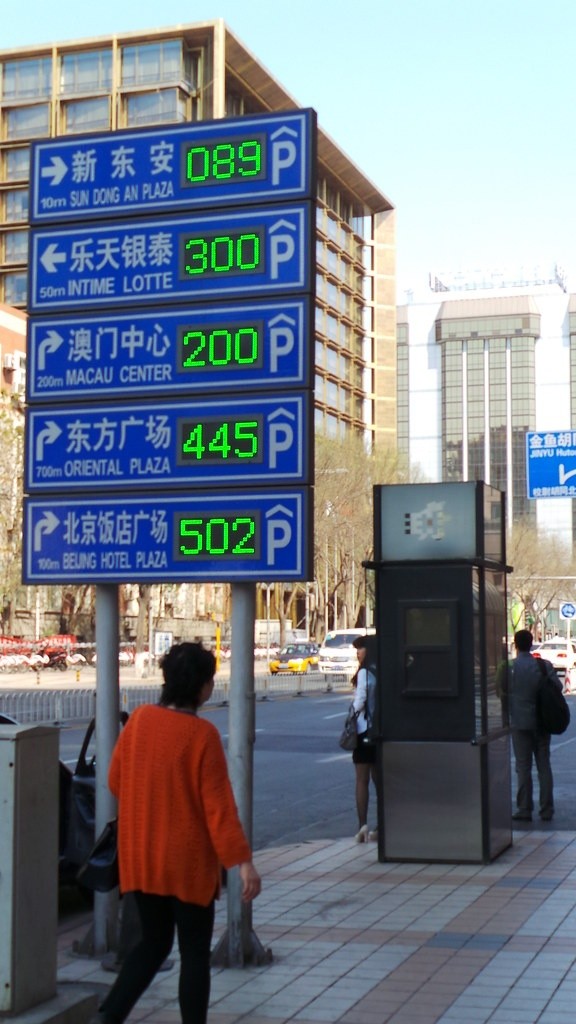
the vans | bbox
[319,628,378,681]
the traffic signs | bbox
[22,108,316,583]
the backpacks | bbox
[532,657,570,736]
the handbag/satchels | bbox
[76,816,119,892]
[338,703,357,750]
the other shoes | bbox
[511,809,533,822]
[538,809,553,822]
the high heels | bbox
[368,829,378,841]
[354,824,369,843]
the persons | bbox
[350,635,378,842]
[292,645,301,653]
[97,642,261,1024]
[553,633,559,639]
[495,648,509,727]
[507,630,563,818]
[538,632,541,642]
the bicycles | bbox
[0,643,134,675]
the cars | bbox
[0,712,129,912]
[530,640,576,672]
[269,642,322,675]
